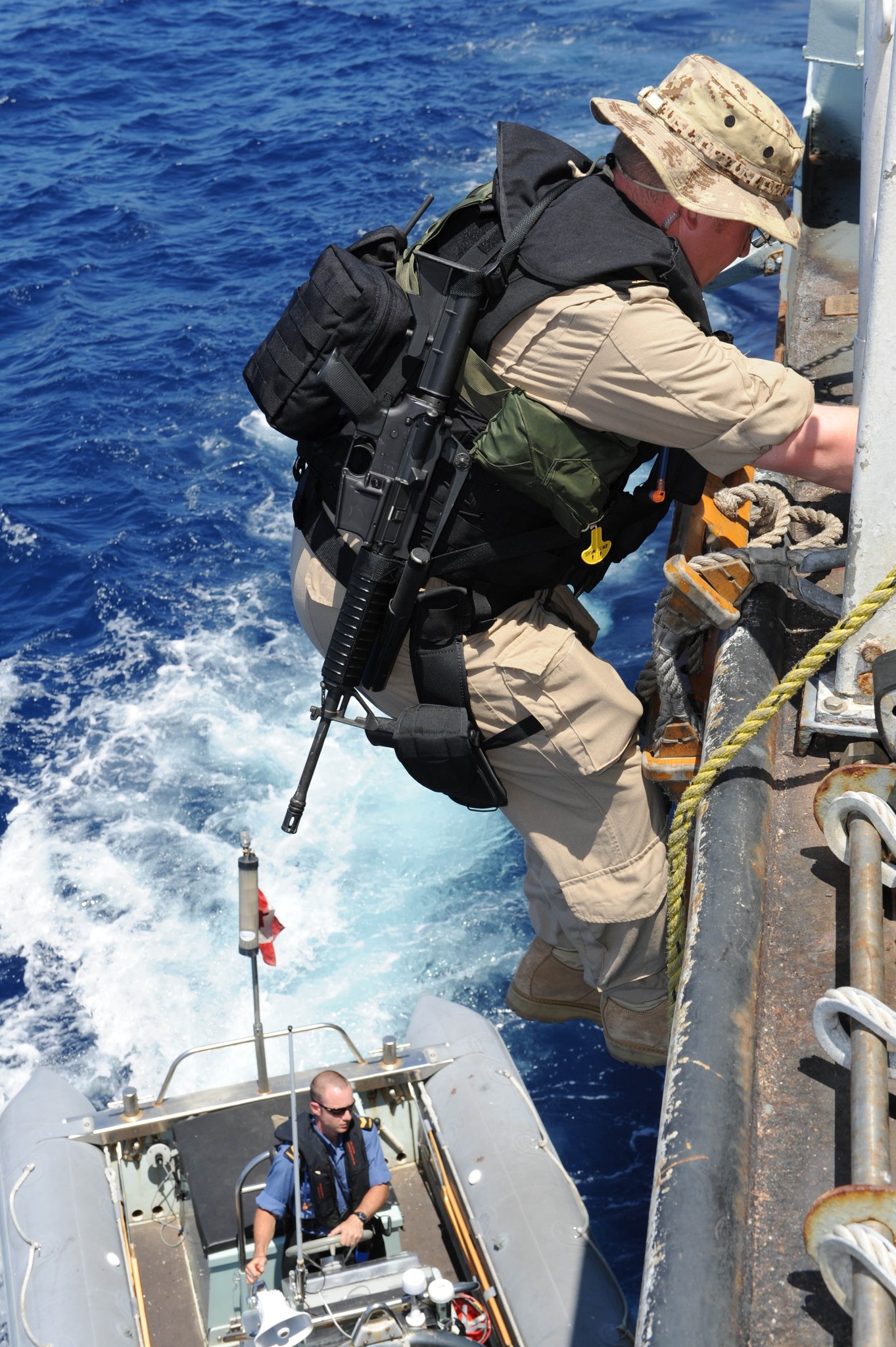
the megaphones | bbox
[252,1288,314,1347]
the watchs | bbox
[353,1212,367,1224]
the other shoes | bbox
[598,997,668,1066]
[505,936,601,1026]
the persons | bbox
[289,53,865,1067]
[242,1069,389,1289]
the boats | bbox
[0,830,637,1347]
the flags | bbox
[258,890,286,966]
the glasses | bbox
[749,226,771,248]
[312,1097,355,1116]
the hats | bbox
[589,52,806,248]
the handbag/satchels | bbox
[242,243,415,442]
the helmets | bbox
[435,1292,491,1345]
